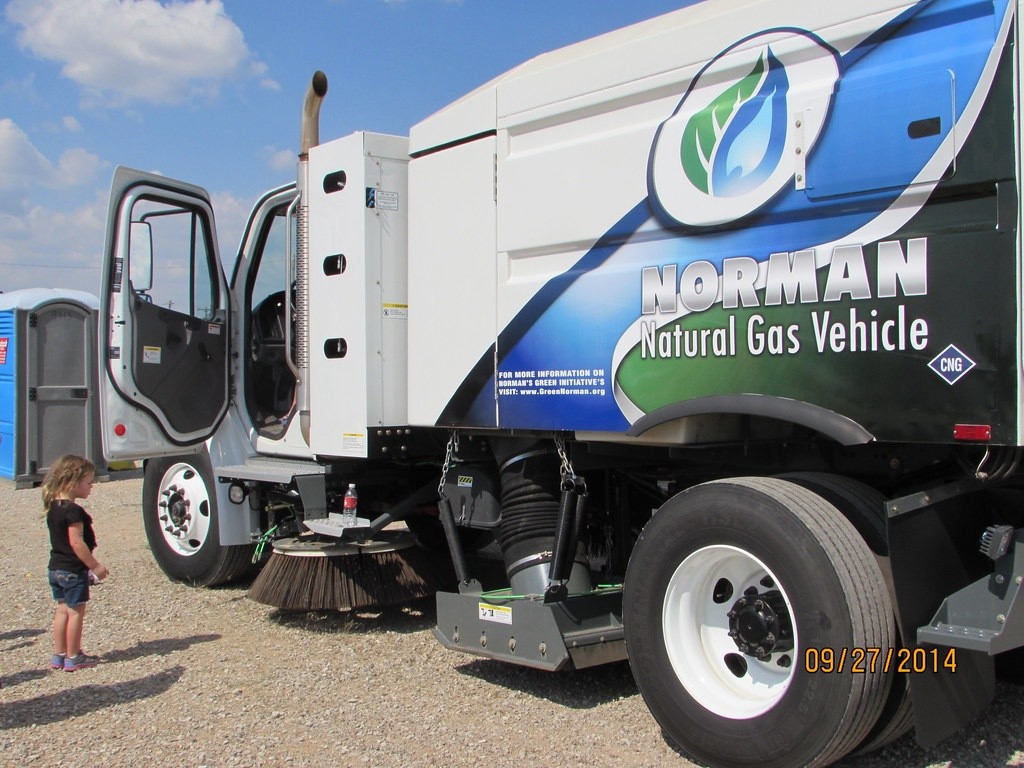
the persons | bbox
[41,454,110,671]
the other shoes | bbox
[280,415,288,425]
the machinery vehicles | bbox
[97,1,1023,768]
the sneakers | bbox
[64,649,99,671]
[51,654,67,669]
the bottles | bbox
[343,484,358,527]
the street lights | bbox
[197,307,211,319]
